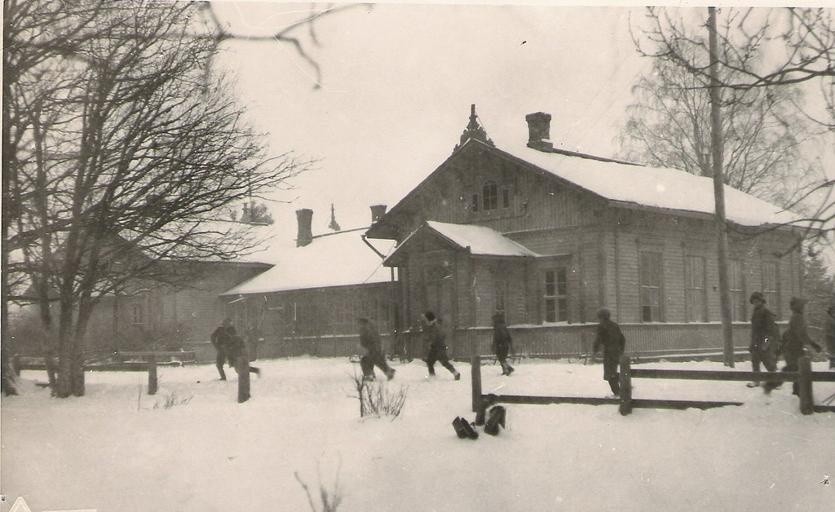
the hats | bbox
[748,291,768,305]
[789,296,810,314]
[420,312,437,326]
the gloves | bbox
[811,342,822,354]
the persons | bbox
[210,317,235,381]
[492,313,514,377]
[421,310,461,380]
[746,291,780,388]
[823,303,835,371]
[761,296,823,396]
[357,316,396,382]
[224,326,261,378]
[245,320,260,362]
[592,308,627,395]
[10,352,22,376]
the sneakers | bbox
[746,381,760,388]
[500,365,514,377]
